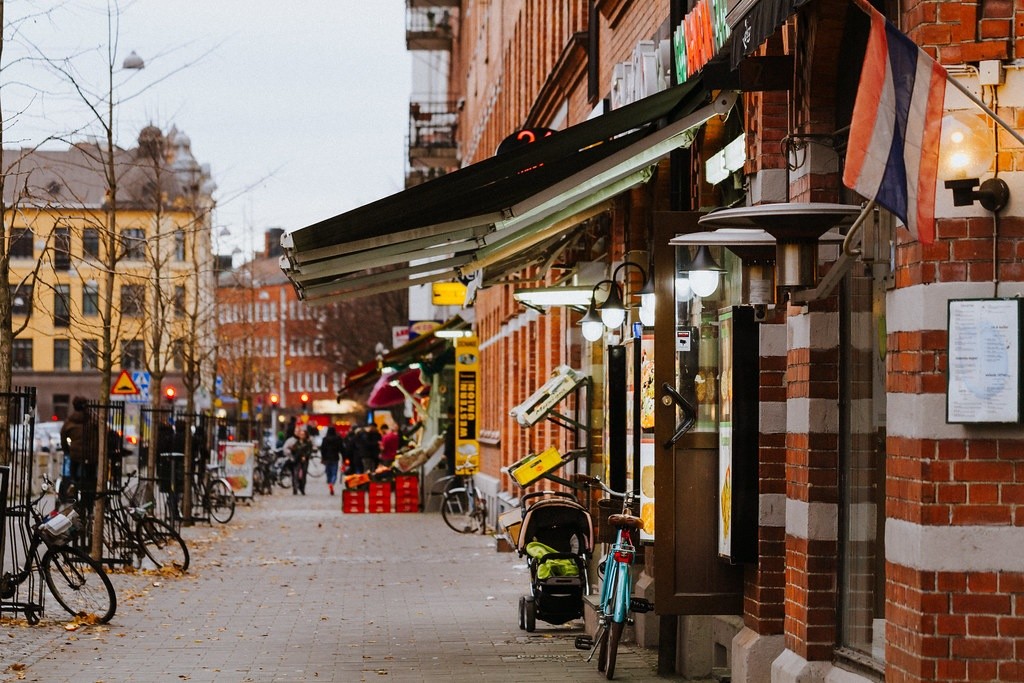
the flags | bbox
[842,7,947,244]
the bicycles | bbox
[442,452,487,535]
[576,472,645,677]
[182,458,235,525]
[87,469,189,574]
[253,443,325,497]
[0,472,118,626]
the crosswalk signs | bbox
[126,370,155,403]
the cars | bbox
[10,420,70,452]
[104,422,140,457]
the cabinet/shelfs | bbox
[516,375,593,505]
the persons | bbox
[156,417,207,494]
[61,396,121,513]
[277,416,422,496]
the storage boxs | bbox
[498,365,577,546]
[341,437,444,514]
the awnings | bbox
[280,77,741,307]
[344,316,467,399]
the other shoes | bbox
[293,486,305,496]
[328,483,334,496]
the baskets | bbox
[120,480,156,515]
[597,498,640,545]
[31,498,80,551]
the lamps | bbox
[668,227,868,319]
[926,110,1010,211]
[478,302,542,352]
[675,244,696,302]
[513,285,612,308]
[674,208,742,301]
[575,258,655,343]
[699,191,880,312]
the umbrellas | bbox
[367,369,430,418]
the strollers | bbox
[516,491,592,632]
